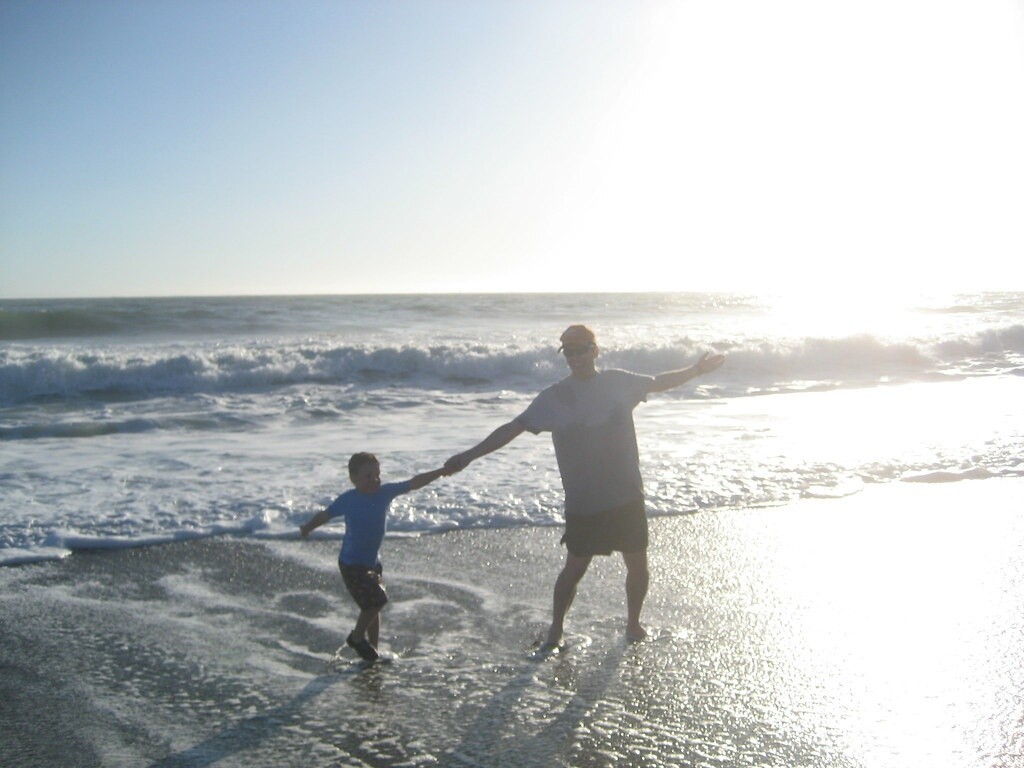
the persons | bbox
[300,452,461,662]
[444,325,726,651]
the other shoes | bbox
[346,630,379,661]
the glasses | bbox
[563,344,592,358]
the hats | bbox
[557,324,594,355]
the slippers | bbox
[626,627,647,642]
[546,638,566,652]
